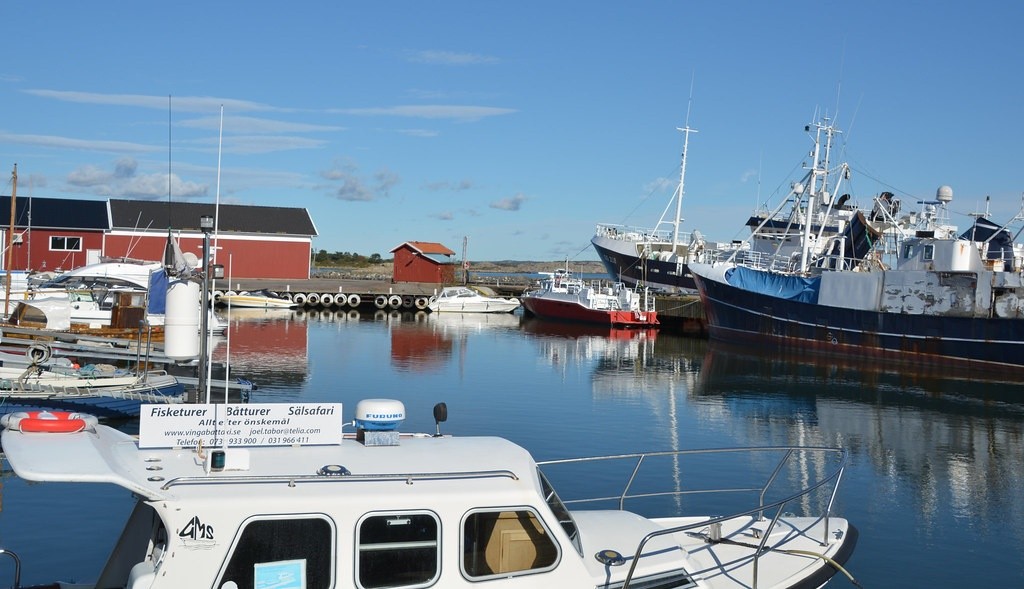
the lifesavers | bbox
[510,298,520,304]
[415,297,428,309]
[402,296,414,308]
[348,293,361,307]
[334,294,348,306]
[429,296,440,304]
[293,293,306,308]
[211,291,224,304]
[0,409,101,434]
[226,291,238,298]
[389,296,402,309]
[271,291,278,297]
[293,308,428,320]
[199,291,211,302]
[321,293,334,307]
[375,295,388,309]
[306,293,320,306]
[239,291,251,296]
[279,292,292,302]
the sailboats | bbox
[1,87,260,429]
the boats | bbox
[517,310,660,385]
[217,295,299,309]
[518,245,660,329]
[0,397,862,589]
[685,327,1024,426]
[425,310,520,333]
[426,286,521,314]
[221,304,296,324]
[591,80,1023,379]
